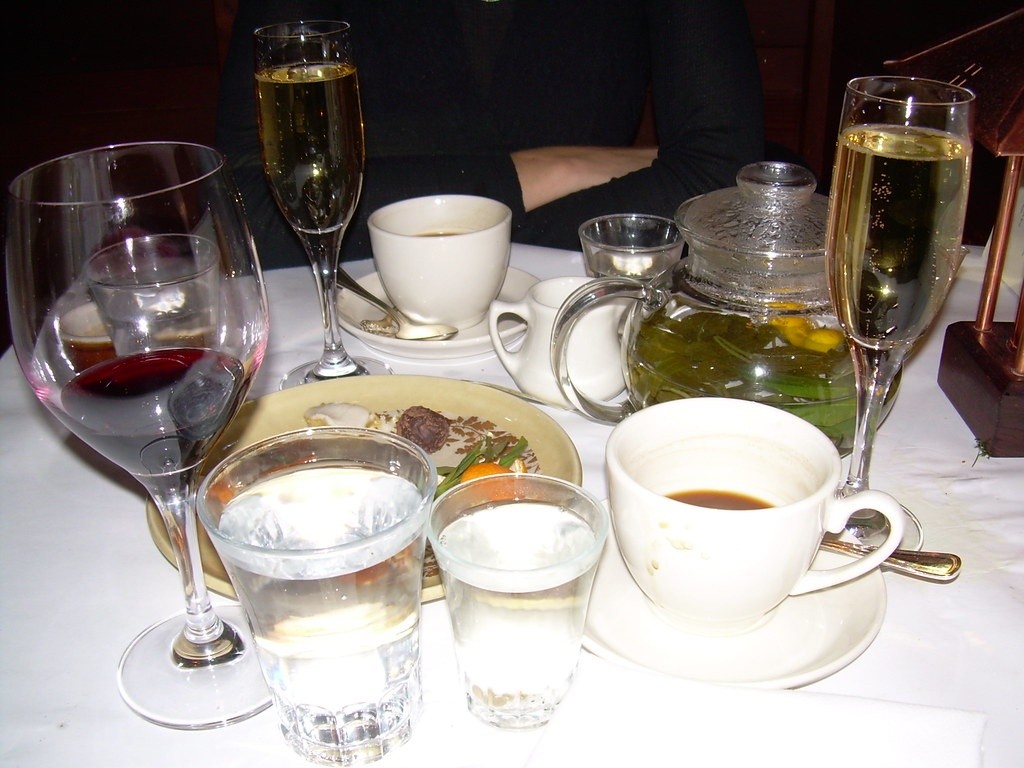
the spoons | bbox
[360,305,400,336]
[336,265,459,341]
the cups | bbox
[86,232,221,355]
[605,398,904,623]
[54,282,221,374]
[426,473,609,732]
[368,194,513,330]
[578,213,683,334]
[195,424,439,768]
[488,277,628,410]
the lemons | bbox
[762,299,845,353]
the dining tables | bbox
[0,241,1024,768]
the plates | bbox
[582,496,886,690]
[336,266,542,360]
[144,372,582,605]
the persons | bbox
[208,1,767,277]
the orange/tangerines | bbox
[458,458,530,500]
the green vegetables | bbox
[433,436,534,500]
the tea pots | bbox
[551,158,971,460]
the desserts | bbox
[397,405,449,454]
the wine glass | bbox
[825,75,977,571]
[254,19,393,393]
[4,140,273,730]
